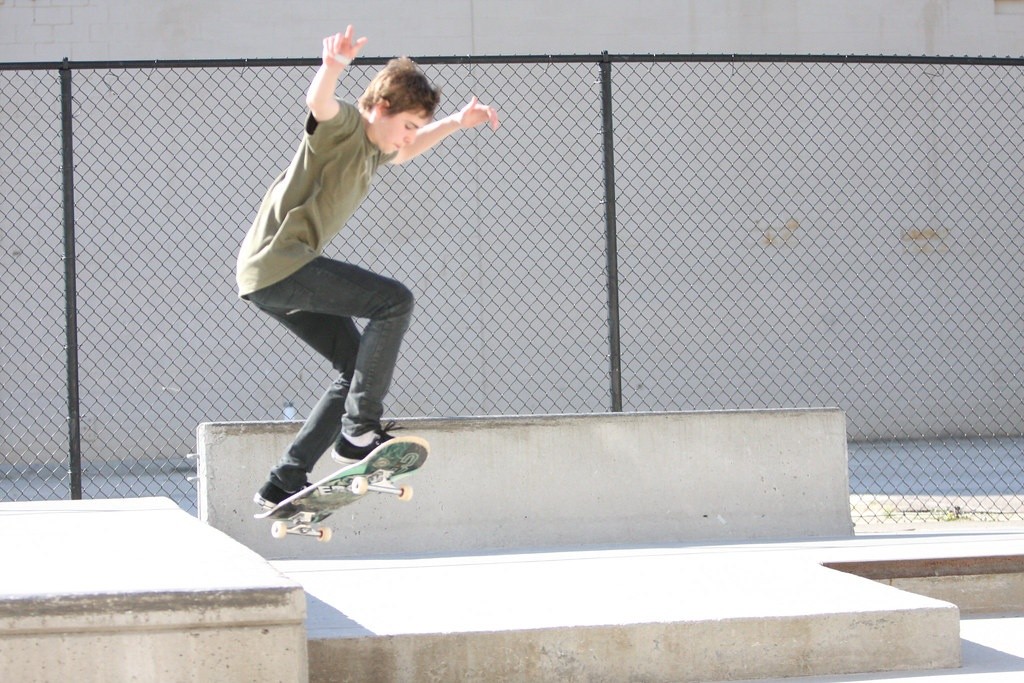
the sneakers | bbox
[332,427,396,466]
[253,480,316,512]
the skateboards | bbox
[254,435,430,540]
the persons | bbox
[231,23,497,512]
